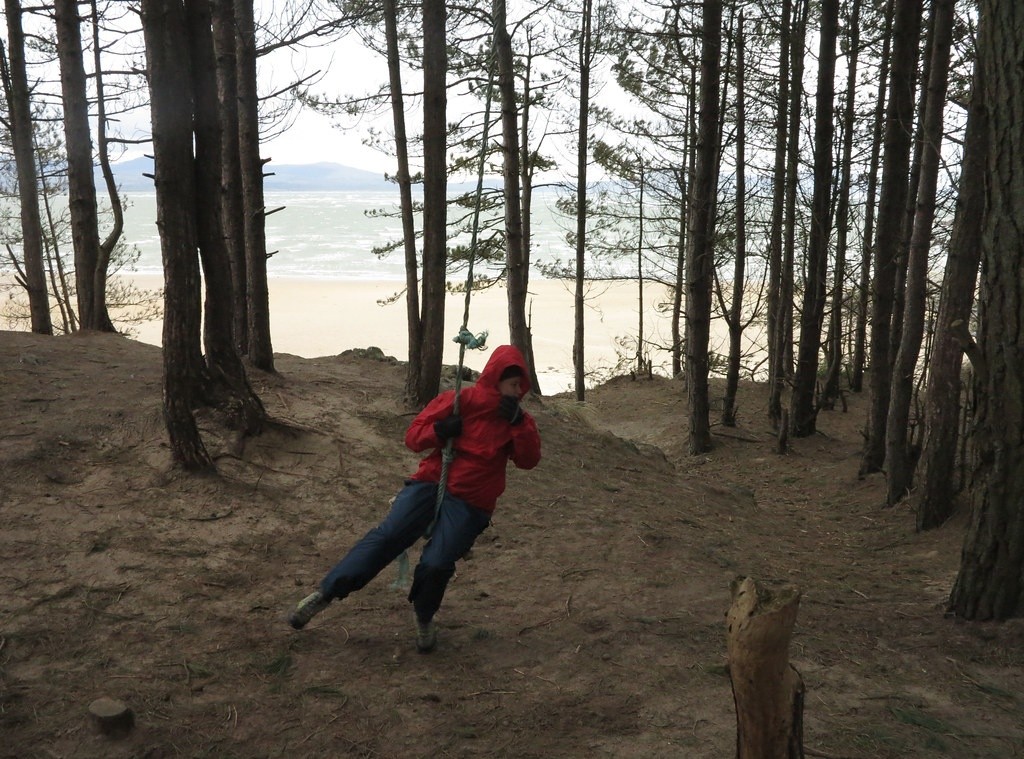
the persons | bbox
[288,344,541,654]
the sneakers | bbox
[413,611,437,654]
[288,590,327,630]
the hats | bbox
[498,366,524,382]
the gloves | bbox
[497,396,524,426]
[433,414,463,441]
